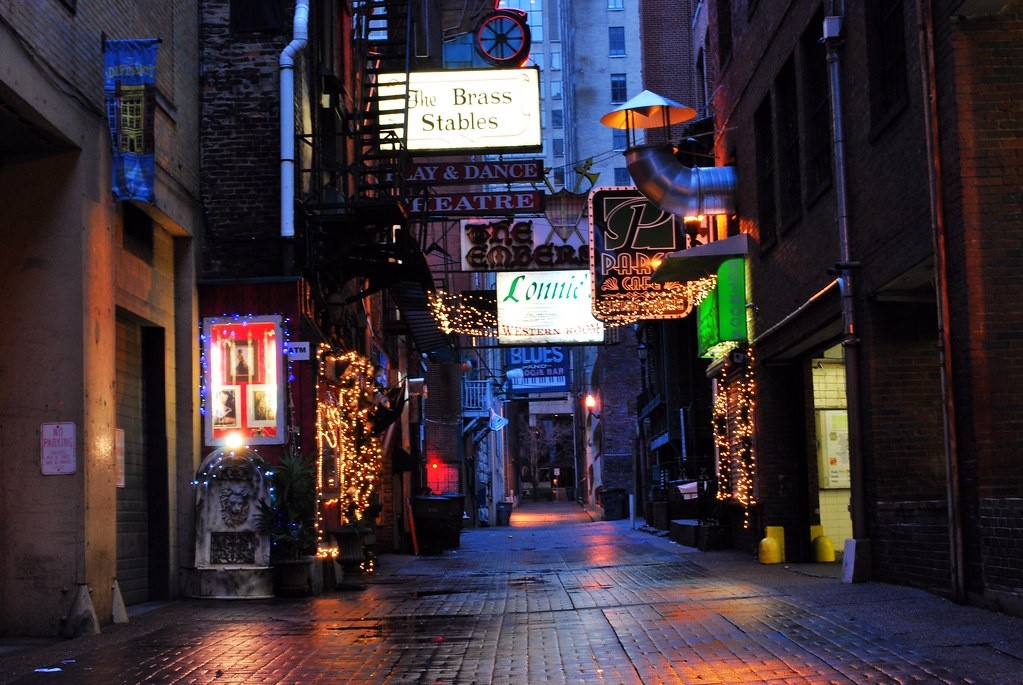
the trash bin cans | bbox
[413,495,452,555]
[442,491,466,550]
[496,501,514,527]
[598,488,623,520]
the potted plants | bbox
[253,450,323,597]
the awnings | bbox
[649,231,748,285]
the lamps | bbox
[682,215,704,248]
[584,393,599,420]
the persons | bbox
[236,349,248,373]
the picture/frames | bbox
[244,384,276,428]
[225,340,260,382]
[212,385,240,428]
